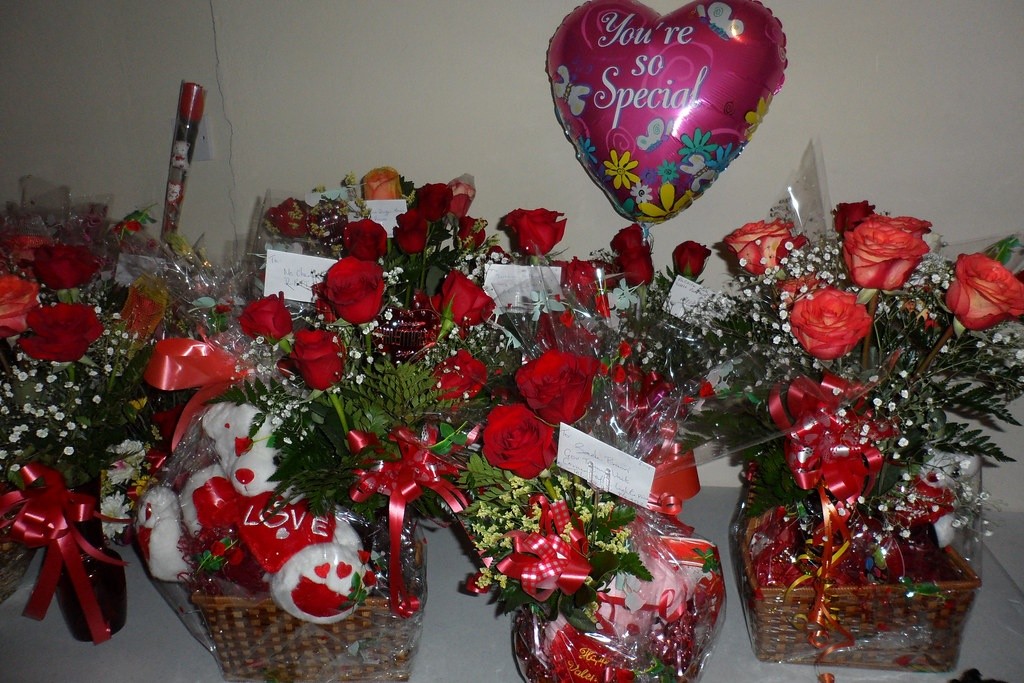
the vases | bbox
[0,514,39,601]
[50,481,129,646]
[192,502,441,679]
[728,519,984,670]
[504,539,718,679]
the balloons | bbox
[545,0,789,229]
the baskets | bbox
[0,502,978,683]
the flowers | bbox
[0,172,1024,635]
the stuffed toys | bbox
[139,399,376,624]
[168,179,182,205]
[891,444,981,583]
[544,542,713,664]
[172,140,190,169]
